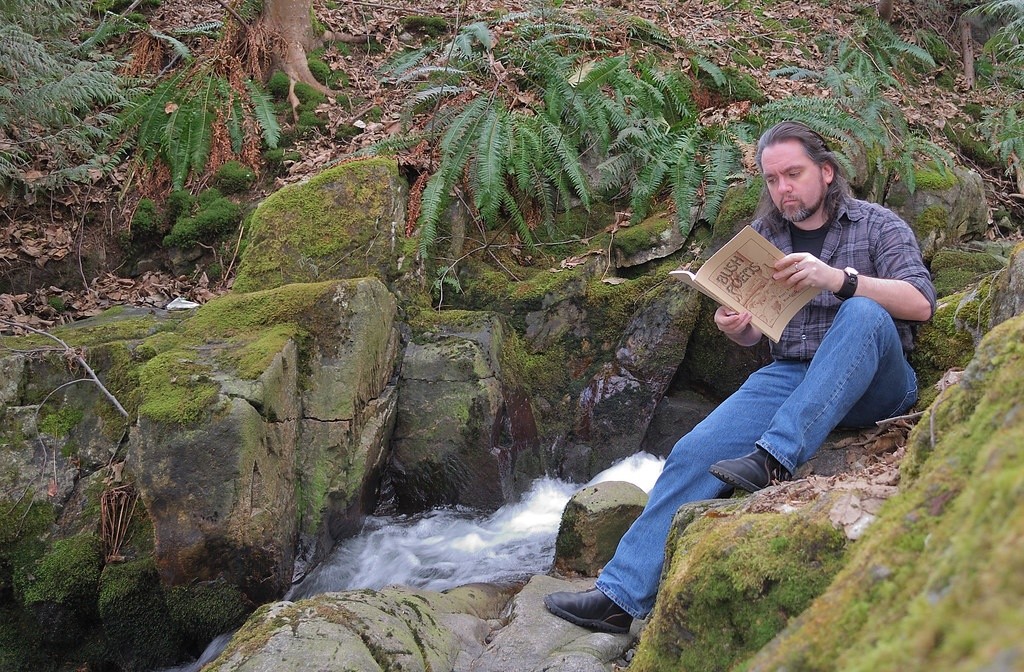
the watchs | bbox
[832,267,859,301]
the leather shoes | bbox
[544,588,634,633]
[708,447,792,494]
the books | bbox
[669,224,821,344]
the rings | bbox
[795,263,799,272]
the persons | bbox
[544,120,936,634]
